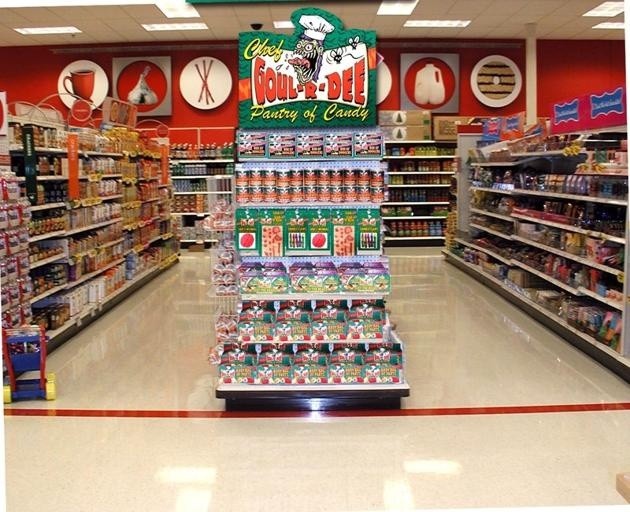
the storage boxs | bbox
[377,109,492,142]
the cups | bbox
[64,67,96,105]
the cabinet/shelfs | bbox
[1,114,184,413]
[378,140,459,248]
[207,8,414,408]
[167,142,235,248]
[441,112,629,375]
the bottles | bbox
[170,141,234,174]
[388,160,453,202]
[387,220,444,237]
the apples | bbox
[241,234,253,247]
[312,234,325,247]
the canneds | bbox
[235,167,384,204]
[32,301,70,331]
